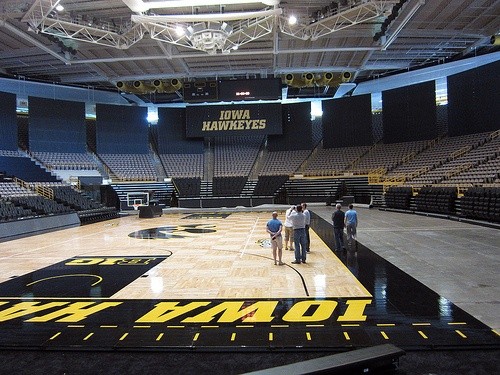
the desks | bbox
[138,205,166,218]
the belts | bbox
[294,228,305,230]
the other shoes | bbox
[274,262,277,265]
[289,248,294,251]
[335,248,338,251]
[342,246,346,250]
[307,250,310,253]
[300,259,305,263]
[285,247,288,250]
[291,260,301,264]
[279,262,285,265]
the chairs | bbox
[0,134,500,221]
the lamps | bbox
[82,14,88,22]
[93,17,100,25]
[56,3,64,11]
[47,10,57,18]
[39,30,77,56]
[70,10,79,18]
[308,0,361,18]
[281,71,353,88]
[116,78,184,93]
[490,35,500,46]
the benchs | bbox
[243,344,405,375]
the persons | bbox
[301,203,310,253]
[266,212,284,265]
[284,209,296,250]
[331,205,345,251]
[287,205,306,264]
[344,205,358,243]
